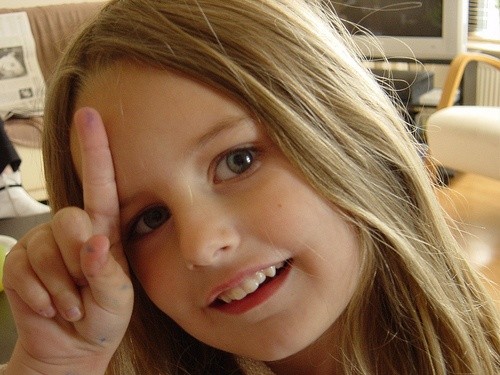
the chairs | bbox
[423,51,500,321]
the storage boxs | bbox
[367,69,435,105]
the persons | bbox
[0,0,500,375]
[0,118,52,221]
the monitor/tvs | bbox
[318,1,468,64]
